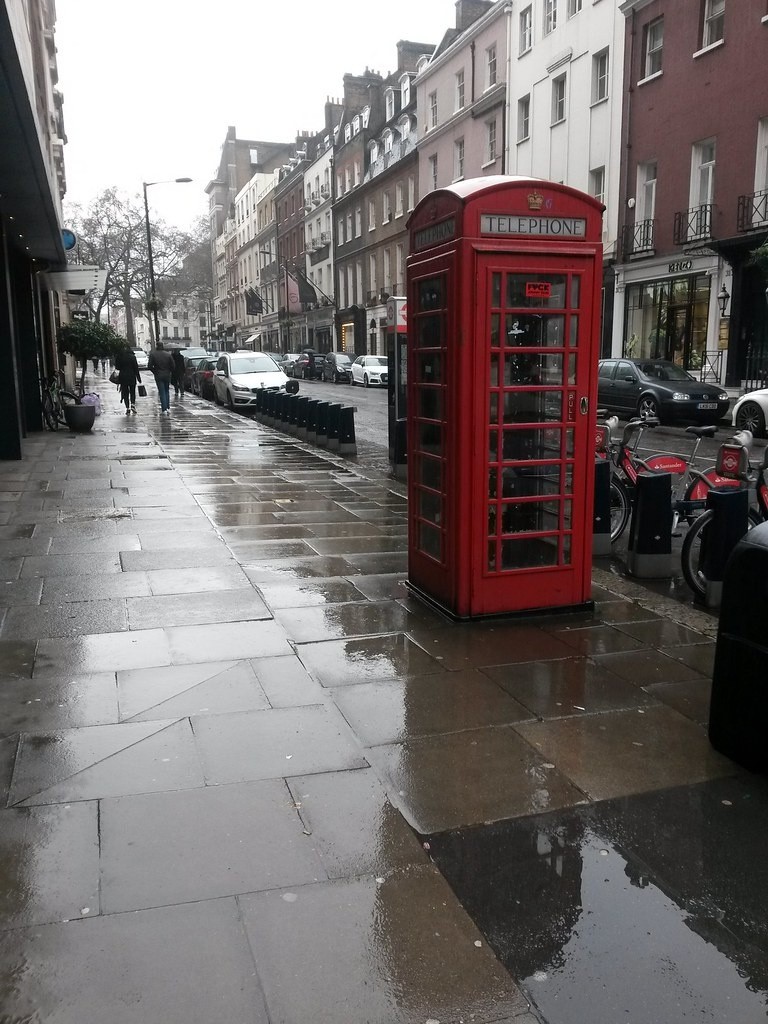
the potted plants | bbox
[55,320,131,433]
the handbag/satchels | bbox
[138,385,147,396]
[109,369,120,384]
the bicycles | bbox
[41,369,83,431]
[543,411,768,597]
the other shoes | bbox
[131,405,137,413]
[161,411,167,416]
[167,409,171,414]
[125,410,131,415]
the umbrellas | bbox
[163,343,188,354]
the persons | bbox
[306,353,317,381]
[115,344,142,415]
[170,348,186,398]
[148,342,175,415]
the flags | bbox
[246,268,318,316]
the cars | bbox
[322,352,357,384]
[732,389,768,435]
[133,351,149,368]
[350,356,388,388]
[267,351,327,380]
[598,359,729,423]
[173,346,218,400]
[214,352,290,408]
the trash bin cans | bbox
[706,520,768,756]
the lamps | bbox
[716,282,730,317]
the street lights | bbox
[143,178,193,344]
